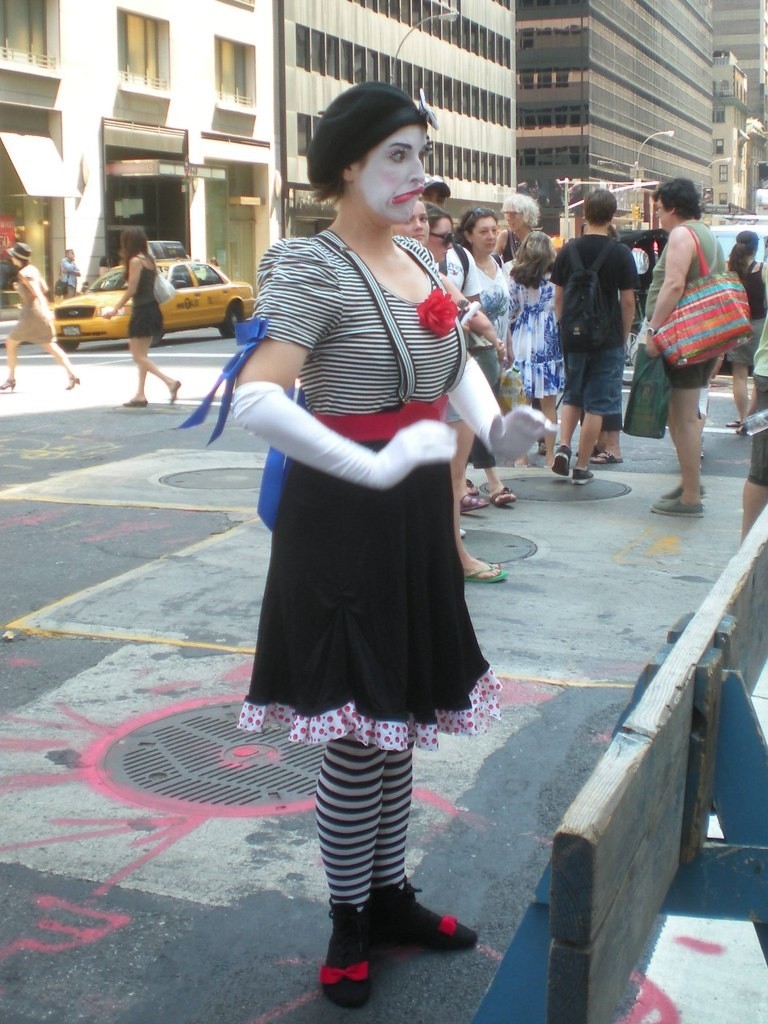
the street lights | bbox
[389,12,461,89]
[634,131,673,181]
[695,156,732,216]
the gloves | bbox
[446,355,561,462]
[230,380,456,490]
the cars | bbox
[53,240,257,353]
[709,224,768,272]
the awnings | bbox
[0,132,82,198]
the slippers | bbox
[726,418,743,426]
[464,562,508,582]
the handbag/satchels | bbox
[152,271,176,304]
[651,226,753,368]
[622,342,672,439]
[54,280,69,295]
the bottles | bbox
[741,408,768,436]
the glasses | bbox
[430,230,454,248]
[463,207,497,228]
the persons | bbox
[228,82,558,1008]
[0,242,81,392]
[60,249,80,299]
[102,226,182,407]
[393,176,768,584]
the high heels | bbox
[0,375,16,390]
[65,376,81,390]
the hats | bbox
[6,242,32,260]
[421,175,451,196]
[306,79,441,192]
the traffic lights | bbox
[634,207,641,218]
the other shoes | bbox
[171,381,181,404]
[649,494,704,518]
[368,874,476,952]
[124,399,148,408]
[318,894,377,1006]
[460,441,625,513]
[662,483,707,499]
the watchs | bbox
[646,328,658,336]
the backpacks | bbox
[561,239,618,352]
